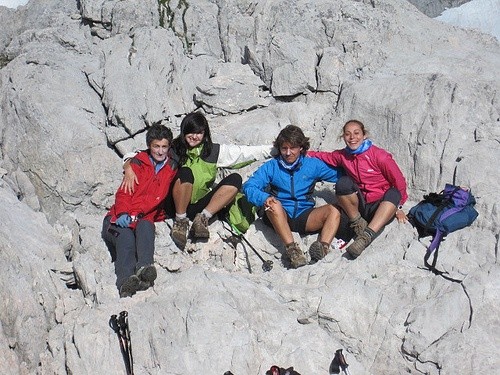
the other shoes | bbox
[120,276,139,296]
[140,266,155,290]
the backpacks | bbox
[415,188,478,233]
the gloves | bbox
[116,214,131,228]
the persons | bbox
[101,123,178,298]
[305,119,408,258]
[242,125,347,267]
[119,112,309,248]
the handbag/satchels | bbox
[227,193,255,232]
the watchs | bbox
[397,205,403,211]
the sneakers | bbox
[347,234,371,258]
[309,241,328,264]
[172,217,188,246]
[351,217,369,238]
[285,245,305,267]
[192,214,208,237]
